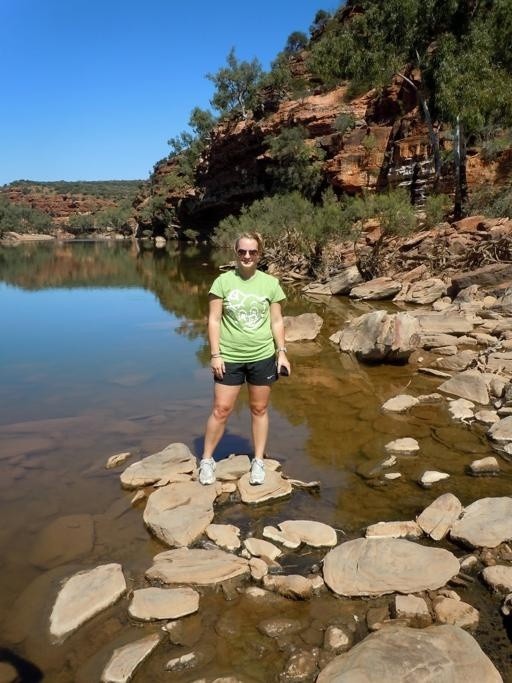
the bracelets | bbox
[276,348,288,353]
[210,352,222,358]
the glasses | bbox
[238,249,258,256]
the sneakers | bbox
[249,457,265,485]
[200,457,216,485]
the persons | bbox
[196,230,292,486]
